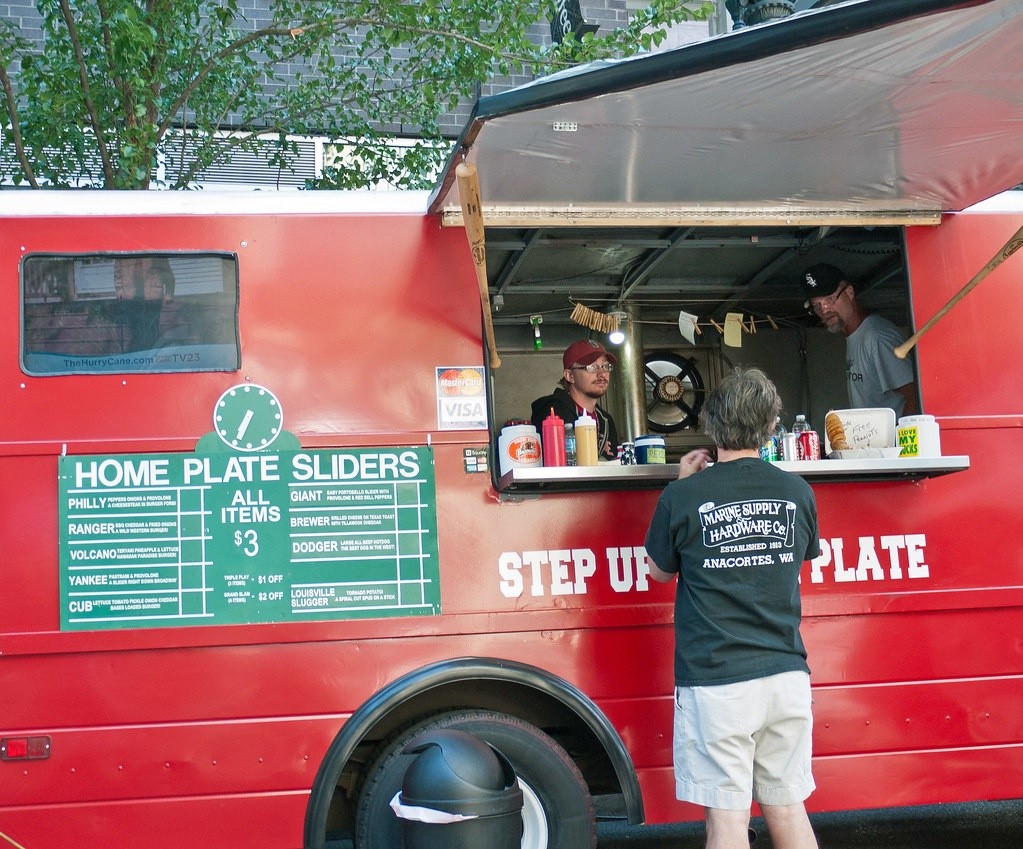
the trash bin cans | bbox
[400,728,524,849]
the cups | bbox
[621,442,637,465]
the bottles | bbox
[895,414,943,458]
[617,445,624,459]
[565,423,577,466]
[792,414,811,434]
[497,418,542,477]
[634,434,666,464]
[575,407,598,466]
[775,416,787,435]
[542,407,566,466]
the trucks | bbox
[0,0,1023,849]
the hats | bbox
[800,262,845,298]
[563,339,617,369]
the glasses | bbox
[813,286,847,314]
[570,364,614,372]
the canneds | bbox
[758,430,821,462]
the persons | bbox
[531,339,618,460]
[644,370,820,849]
[802,263,916,419]
[114,258,174,352]
